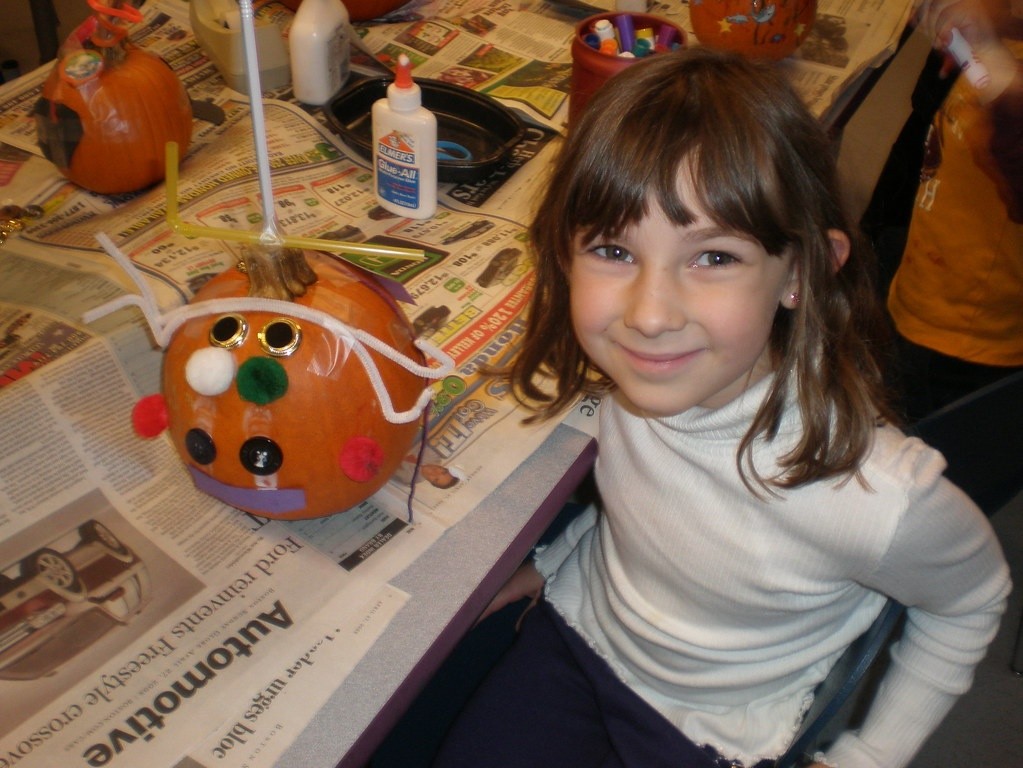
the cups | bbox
[567,11,688,144]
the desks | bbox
[0,0,925,768]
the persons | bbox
[393,441,460,489]
[436,49,1013,768]
[858,2,1023,422]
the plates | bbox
[322,76,526,180]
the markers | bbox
[582,15,679,61]
